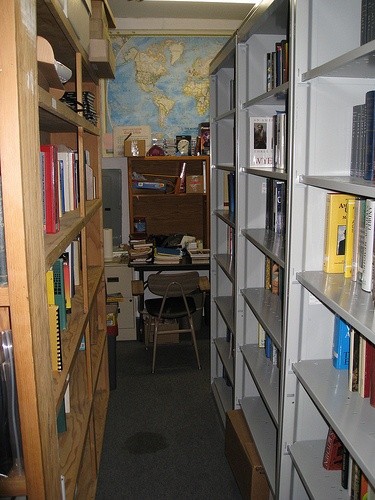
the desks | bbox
[128,250,210,343]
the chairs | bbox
[144,271,202,374]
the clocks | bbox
[175,135,191,156]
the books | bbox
[332,313,375,407]
[107,292,124,327]
[361,0,375,45]
[46,236,81,371]
[61,475,66,500]
[322,426,375,500]
[61,91,97,127]
[226,225,235,256]
[324,193,375,293]
[267,40,289,91]
[223,171,235,216]
[85,151,96,201]
[127,232,211,264]
[264,256,284,300]
[257,322,281,368]
[350,90,375,181]
[39,144,80,234]
[223,365,232,387]
[266,178,286,236]
[230,79,234,110]
[226,326,235,356]
[57,383,71,434]
[250,111,287,169]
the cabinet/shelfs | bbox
[0,0,375,500]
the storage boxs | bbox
[186,174,204,193]
[123,133,146,157]
